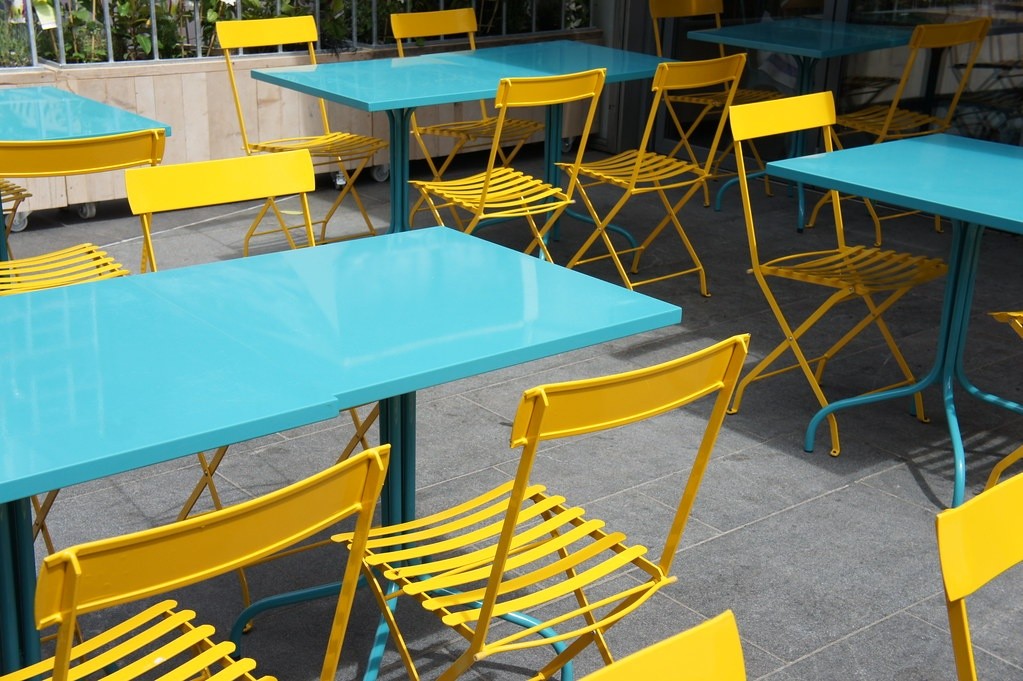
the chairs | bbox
[0,0,1023,681]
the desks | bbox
[250,55,534,235]
[686,15,914,235]
[0,276,338,681]
[764,132,1023,454]
[419,39,683,261]
[125,224,682,681]
[0,85,172,275]
